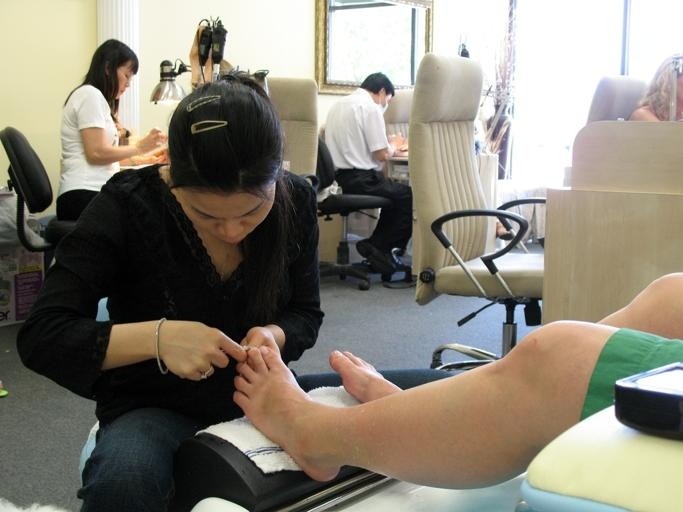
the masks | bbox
[379,95,388,112]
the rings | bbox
[200,365,213,380]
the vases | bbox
[487,105,509,179]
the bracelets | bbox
[154,316,169,374]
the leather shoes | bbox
[356,239,397,274]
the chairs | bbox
[0,125,80,277]
[77,295,110,489]
[382,91,414,183]
[266,78,319,197]
[408,52,547,374]
[316,138,391,290]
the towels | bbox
[195,384,363,474]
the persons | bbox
[54,38,168,223]
[229,270,683,491]
[626,51,683,122]
[325,69,414,270]
[13,69,459,512]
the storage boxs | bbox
[0,246,46,327]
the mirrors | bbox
[315,0,432,95]
